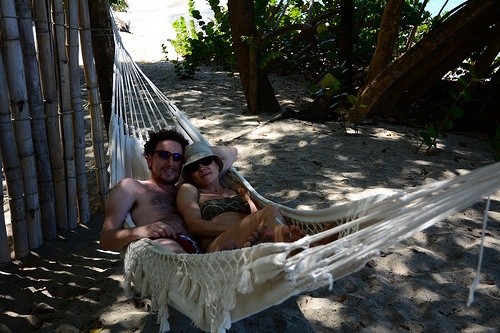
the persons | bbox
[174,141,307,254]
[99,128,273,255]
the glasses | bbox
[188,157,213,171]
[154,150,183,161]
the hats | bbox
[181,140,224,180]
